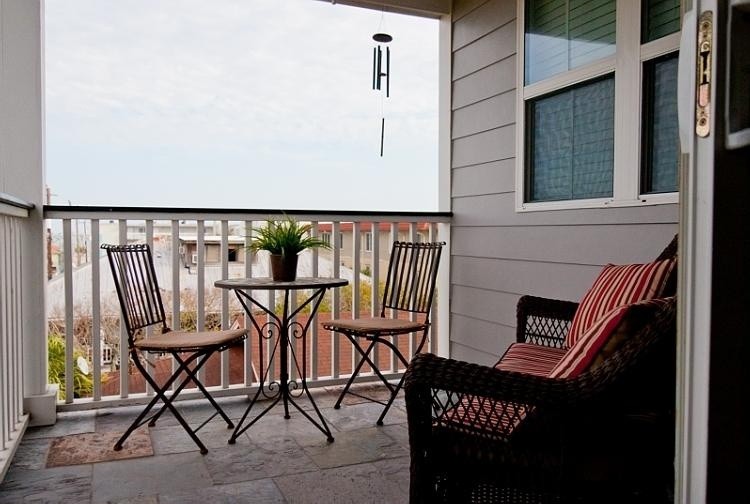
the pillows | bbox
[548,295,677,379]
[561,258,677,351]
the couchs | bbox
[404,233,677,504]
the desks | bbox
[214,277,349,444]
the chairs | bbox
[99,243,251,456]
[320,241,447,425]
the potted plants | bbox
[230,211,335,282]
